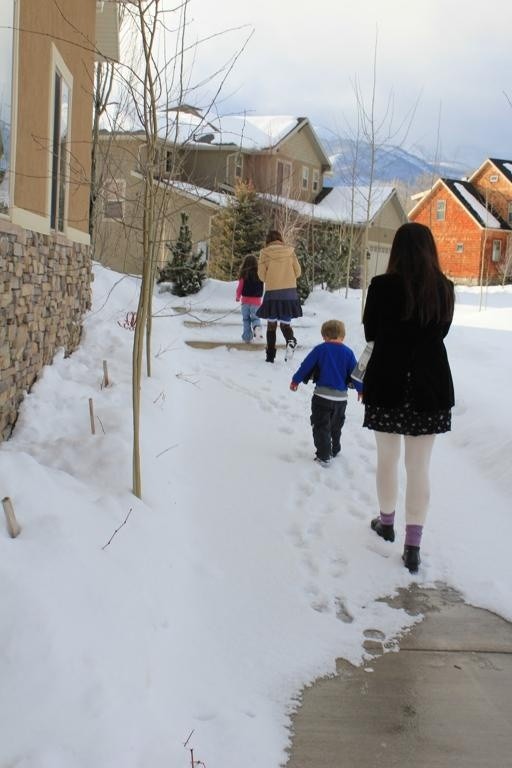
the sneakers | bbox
[254,326,264,341]
[314,457,331,466]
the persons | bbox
[364,222,455,575]
[257,229,301,362]
[290,319,363,463]
[236,255,263,343]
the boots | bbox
[265,347,276,363]
[285,338,297,362]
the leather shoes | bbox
[402,545,421,571]
[371,519,394,541]
